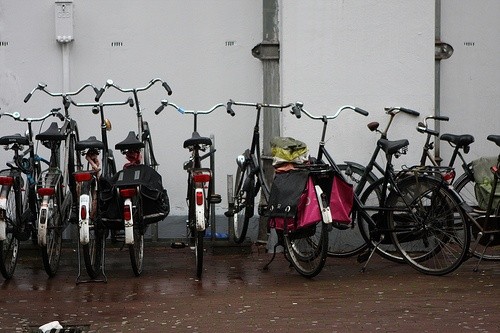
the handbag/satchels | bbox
[472,158,500,210]
[264,167,353,233]
[97,165,170,230]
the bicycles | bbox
[304,106,500,273]
[0,106,66,280]
[223,101,333,279]
[94,77,173,279]
[290,101,474,278]
[63,94,136,286]
[24,82,100,279]
[155,98,238,281]
